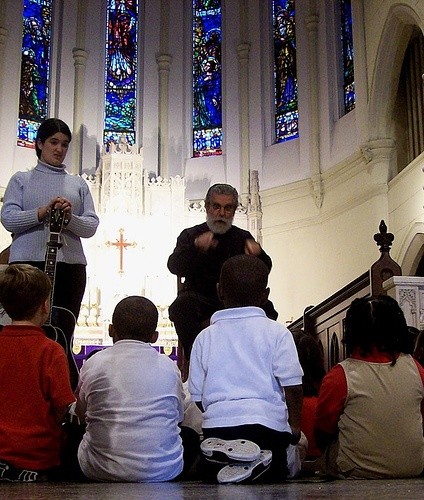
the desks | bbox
[72,344,178,372]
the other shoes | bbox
[217,449,272,484]
[200,437,261,465]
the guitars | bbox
[43,200,81,397]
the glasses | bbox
[212,204,233,213]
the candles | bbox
[94,286,102,305]
[85,275,93,306]
[141,286,145,296]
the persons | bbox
[76,295,186,482]
[405,322,424,383]
[167,184,278,357]
[284,326,330,457]
[315,295,424,480]
[0,264,76,482]
[0,117,100,351]
[190,255,305,483]
